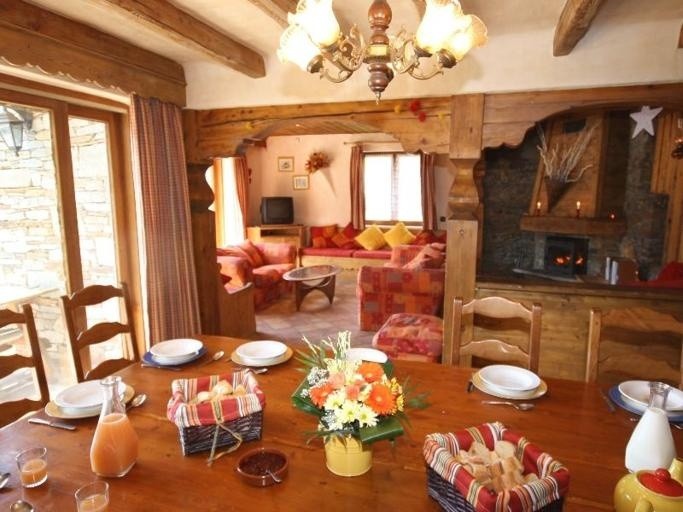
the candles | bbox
[575,201,581,209]
[536,201,541,208]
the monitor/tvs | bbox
[260,196,294,223]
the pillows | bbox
[351,223,386,251]
[382,221,417,250]
[332,221,358,250]
[309,220,339,250]
[411,230,436,244]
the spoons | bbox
[0,472,12,493]
[261,467,283,483]
[125,392,148,412]
[255,368,267,375]
[9,500,35,512]
[195,350,225,370]
[480,400,535,412]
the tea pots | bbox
[611,457,683,512]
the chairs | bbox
[452,292,543,376]
[0,302,50,440]
[54,282,140,389]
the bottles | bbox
[90,375,140,479]
[623,379,678,473]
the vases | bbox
[316,424,373,477]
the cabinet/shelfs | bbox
[245,224,310,248]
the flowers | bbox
[303,150,338,197]
[292,331,423,444]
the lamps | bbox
[0,103,35,157]
[275,0,484,107]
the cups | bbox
[73,480,110,512]
[14,445,49,491]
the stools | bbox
[375,308,444,365]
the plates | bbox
[234,448,289,488]
[469,363,548,400]
[44,379,136,423]
[229,340,294,369]
[606,379,683,423]
[141,338,208,367]
[342,347,388,365]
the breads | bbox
[187,380,247,404]
[454,442,539,494]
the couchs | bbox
[355,240,443,333]
[216,238,299,311]
[300,224,444,277]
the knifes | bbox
[140,364,183,372]
[598,389,617,413]
[28,417,76,433]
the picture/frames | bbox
[276,155,295,173]
[291,173,309,191]
